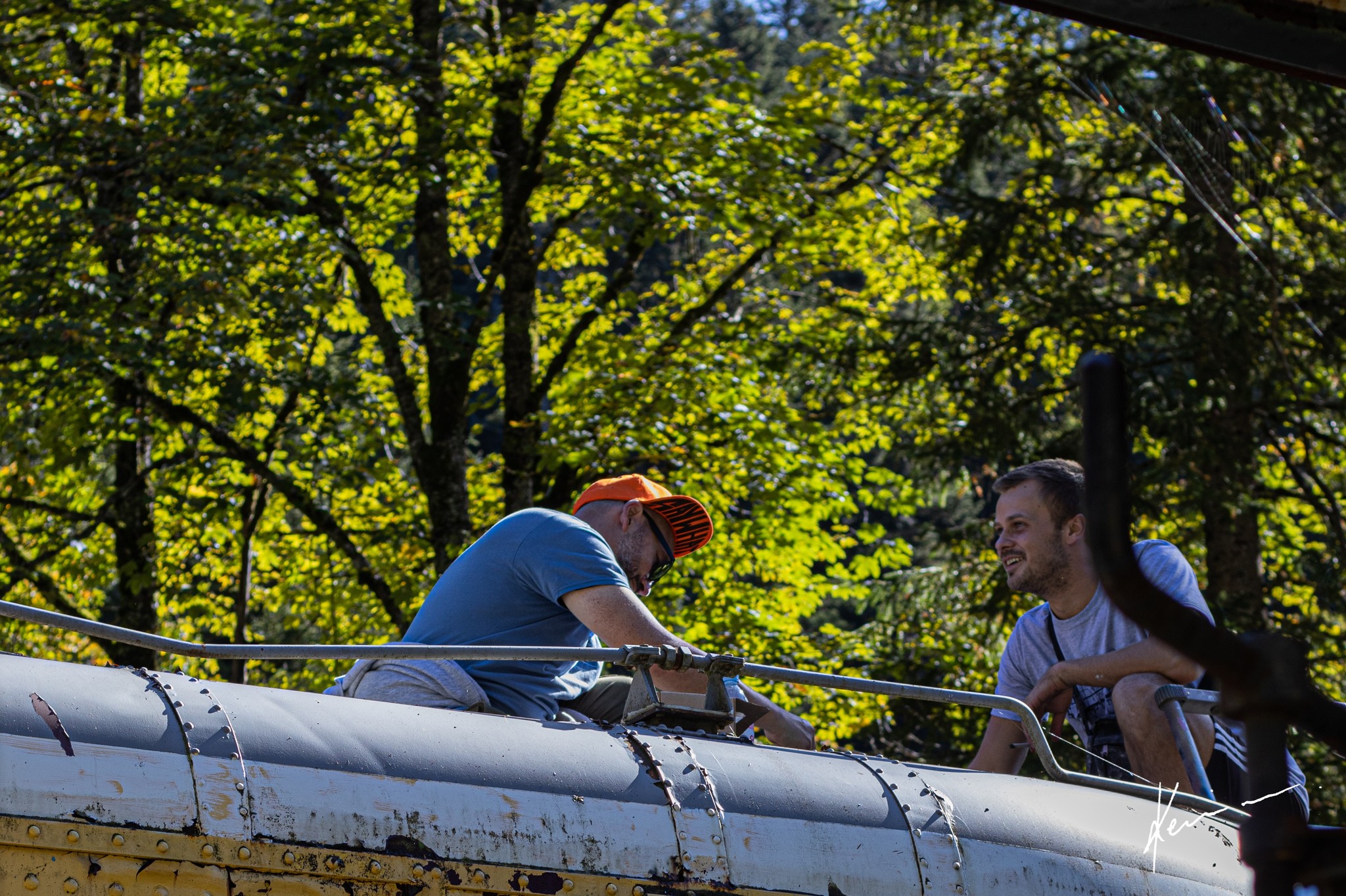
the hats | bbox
[572,473,714,558]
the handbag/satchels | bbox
[1086,716,1135,783]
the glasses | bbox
[623,500,675,588]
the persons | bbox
[322,474,817,752]
[963,458,1313,824]
